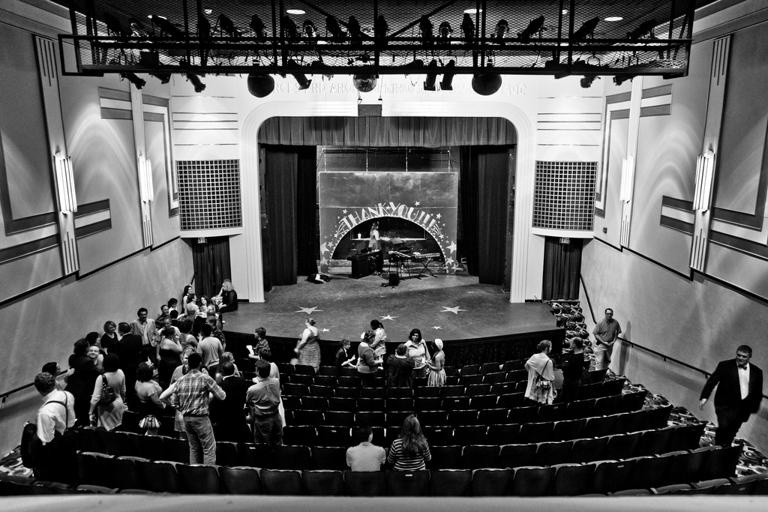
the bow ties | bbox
[738,364,747,370]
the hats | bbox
[435,339,444,350]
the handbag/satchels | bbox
[99,386,116,405]
[540,381,552,390]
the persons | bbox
[550,358,564,390]
[699,345,763,446]
[291,316,447,388]
[524,340,556,405]
[570,336,584,366]
[593,307,621,370]
[368,221,380,251]
[345,415,432,472]
[23,281,287,466]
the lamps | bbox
[620,155,634,203]
[54,154,78,215]
[138,154,154,203]
[692,146,716,215]
